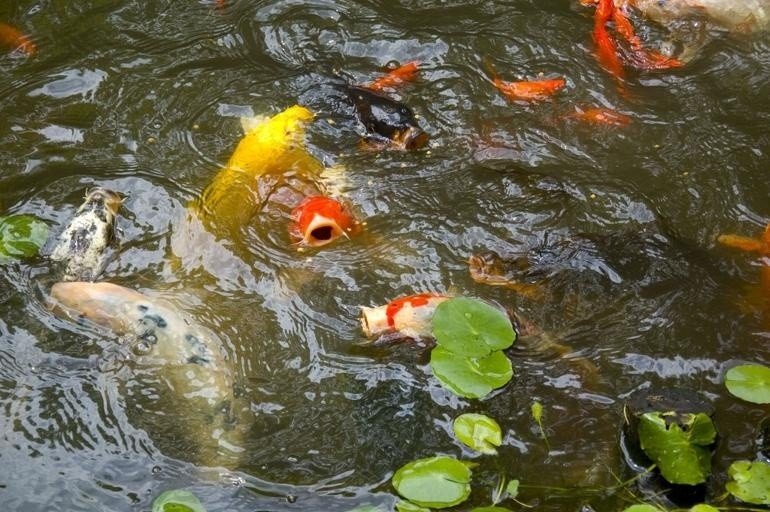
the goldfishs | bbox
[156,61,742,397]
